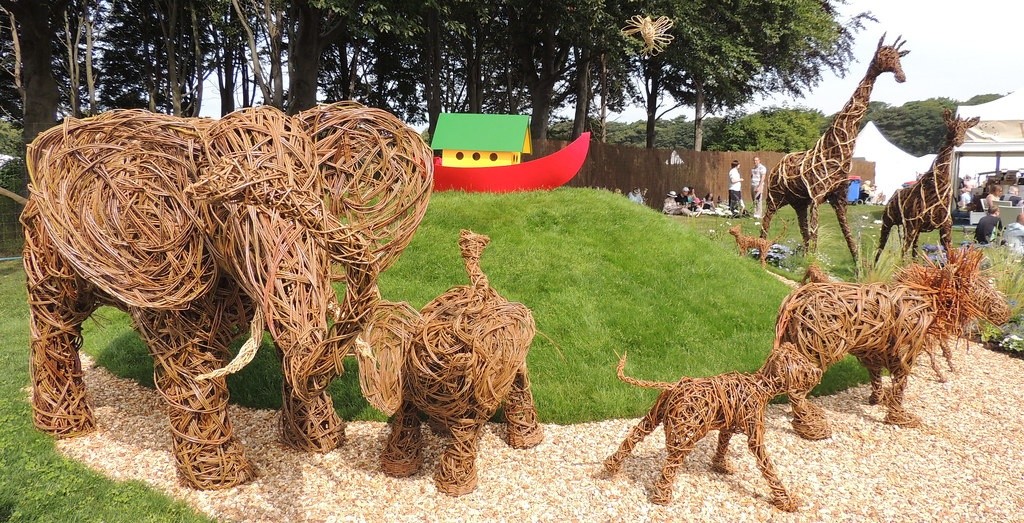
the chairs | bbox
[970,188,1022,229]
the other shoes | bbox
[753,214,761,218]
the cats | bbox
[773,244,983,440]
[728,224,790,267]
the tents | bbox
[951,90,1024,221]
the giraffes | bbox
[870,106,984,272]
[758,29,912,268]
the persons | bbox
[727,160,745,212]
[862,180,871,191]
[664,187,714,217]
[629,188,643,203]
[985,186,1003,208]
[750,157,767,218]
[1000,186,1022,205]
[974,206,1006,243]
[960,185,972,210]
[1003,214,1024,249]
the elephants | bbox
[14,97,436,494]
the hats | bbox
[667,190,677,198]
[681,187,690,192]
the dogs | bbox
[596,342,826,514]
